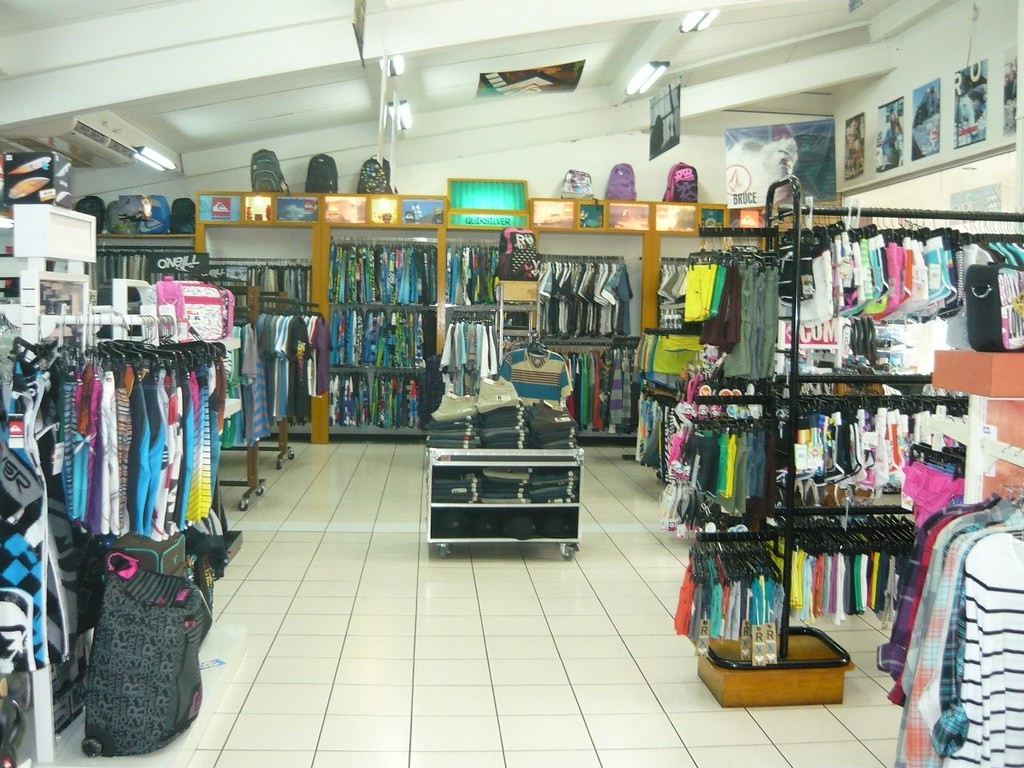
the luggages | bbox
[109,531,188,589]
[80,551,214,759]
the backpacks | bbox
[357,155,398,194]
[250,148,290,193]
[305,154,338,193]
[560,169,594,199]
[76,196,105,234]
[171,198,195,233]
[498,226,541,283]
[663,162,698,203]
[105,201,136,234]
[136,194,170,234]
[605,163,637,201]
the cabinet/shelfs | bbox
[98,231,197,295]
[429,446,585,563]
[193,192,319,446]
[696,174,1024,709]
[320,193,440,448]
[652,201,742,330]
[932,348,1023,511]
[532,202,651,438]
[442,176,534,359]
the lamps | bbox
[640,58,671,98]
[387,100,414,134]
[681,11,719,35]
[134,144,179,177]
[383,57,407,80]
[626,61,655,97]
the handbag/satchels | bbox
[151,275,234,342]
[965,261,1024,352]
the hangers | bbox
[331,234,436,249]
[330,365,427,378]
[659,257,688,266]
[526,329,548,358]
[331,302,437,315]
[642,387,672,407]
[98,242,194,256]
[446,238,500,250]
[696,375,969,437]
[234,305,254,327]
[643,327,697,337]
[614,336,642,351]
[11,310,227,382]
[452,309,527,324]
[225,258,312,269]
[549,339,613,359]
[540,252,625,262]
[689,505,917,589]
[908,440,966,475]
[990,442,1024,509]
[258,296,322,318]
